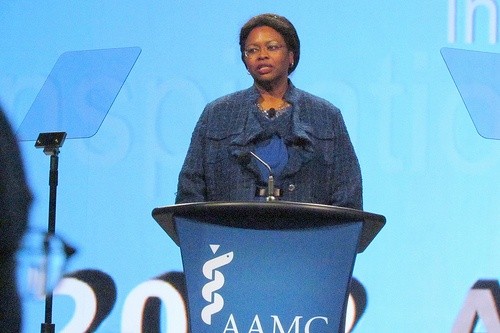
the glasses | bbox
[243,44,289,58]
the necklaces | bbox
[256,100,286,111]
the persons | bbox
[175,14,364,211]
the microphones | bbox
[241,150,275,202]
